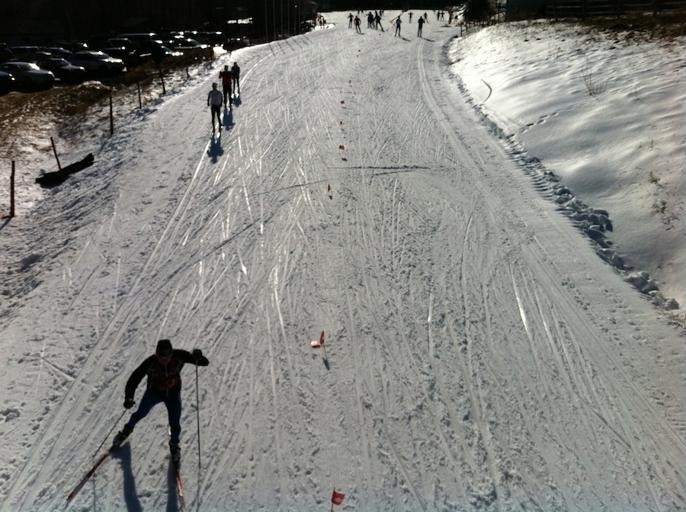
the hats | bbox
[156,339,172,353]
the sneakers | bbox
[113,431,126,448]
[169,440,181,460]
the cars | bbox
[0,29,224,93]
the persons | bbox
[207,62,240,133]
[347,8,458,37]
[114,339,209,461]
[318,15,326,26]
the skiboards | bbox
[66,435,185,512]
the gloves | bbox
[193,350,202,358]
[124,401,135,409]
